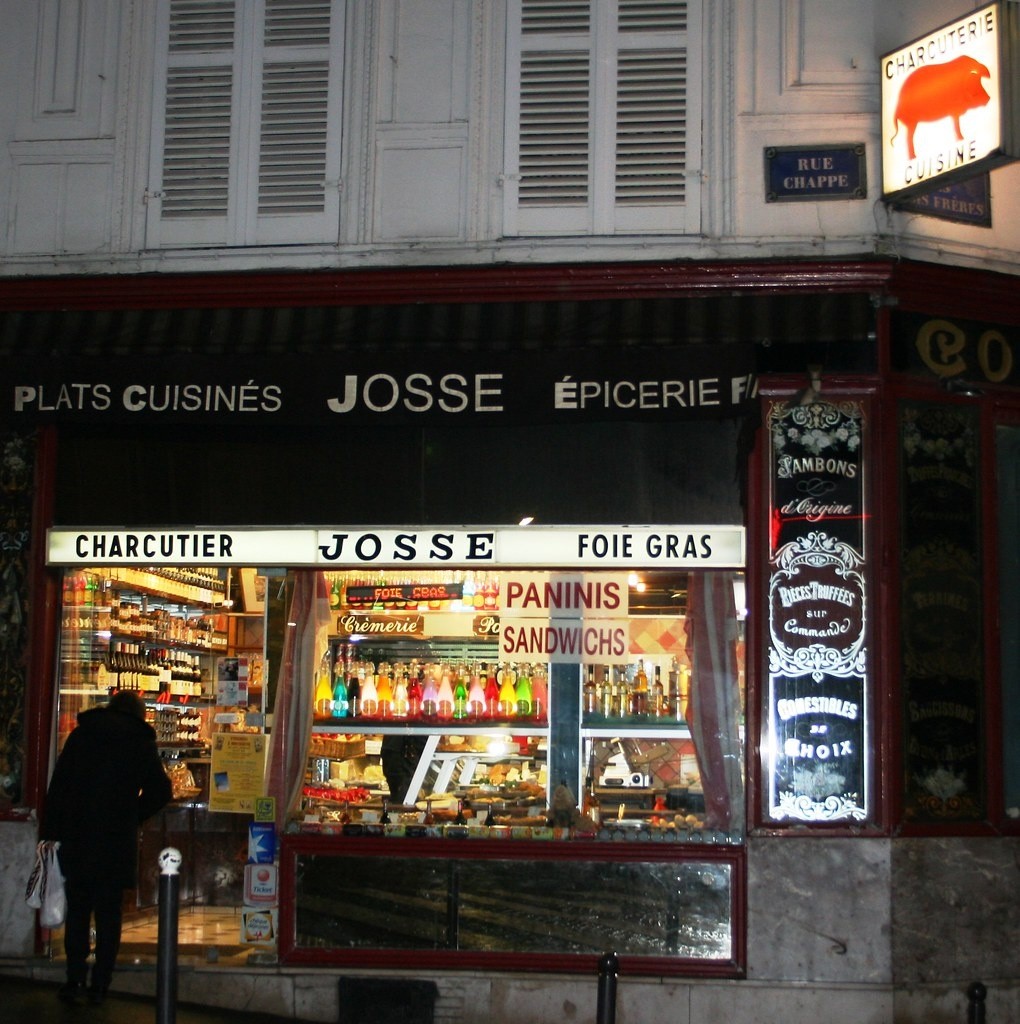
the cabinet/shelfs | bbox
[57,568,267,803]
[309,609,747,815]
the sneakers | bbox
[85,986,107,1003]
[56,982,87,999]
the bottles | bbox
[588,781,601,828]
[305,798,315,815]
[483,801,496,827]
[148,648,202,698]
[170,616,215,647]
[314,660,546,724]
[339,801,350,823]
[61,573,111,607]
[455,800,467,825]
[66,640,147,692]
[379,802,392,824]
[582,656,692,725]
[423,800,435,825]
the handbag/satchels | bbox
[24,840,66,930]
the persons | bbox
[379,732,429,808]
[35,690,174,1008]
